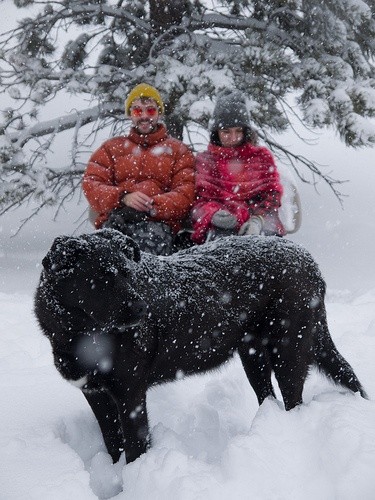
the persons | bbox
[183,84,287,251]
[81,79,195,262]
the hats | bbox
[208,87,251,132]
[124,83,163,115]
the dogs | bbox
[35,228,370,467]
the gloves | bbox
[210,211,238,230]
[238,216,262,236]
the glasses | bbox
[129,105,159,117]
